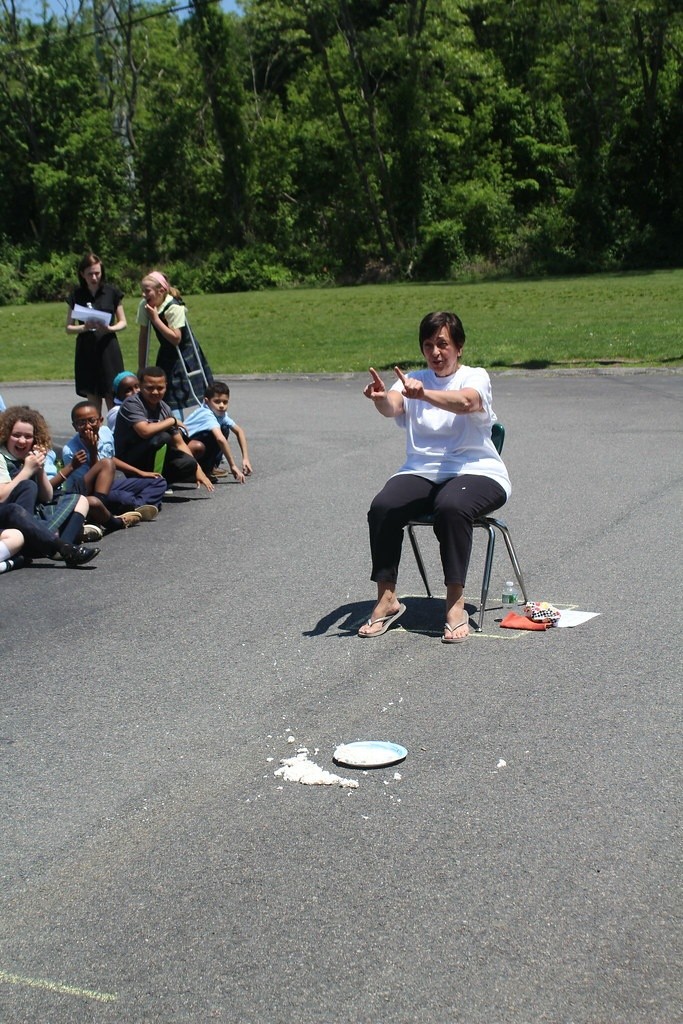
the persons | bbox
[135,272,215,423]
[358,310,512,644]
[0,367,255,575]
[65,252,128,416]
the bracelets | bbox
[59,471,68,482]
[169,416,177,426]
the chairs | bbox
[402,423,528,632]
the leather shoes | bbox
[64,545,101,568]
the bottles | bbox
[502,581,518,620]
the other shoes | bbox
[113,511,142,528]
[80,524,103,542]
[134,505,158,520]
[208,475,218,483]
[213,466,229,477]
[5,555,32,571]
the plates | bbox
[333,740,408,768]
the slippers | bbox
[358,602,406,637]
[441,610,470,643]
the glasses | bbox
[75,417,100,429]
